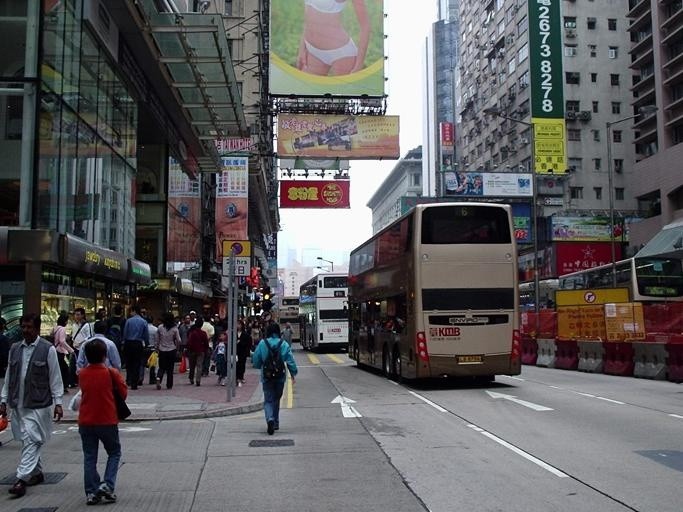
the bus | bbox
[518,255,682,308]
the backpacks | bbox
[263,338,286,382]
[105,316,124,344]
[48,327,62,349]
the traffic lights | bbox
[269,293,275,310]
[0,410,13,447]
[263,286,271,310]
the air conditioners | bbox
[441,1,594,174]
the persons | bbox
[76,337,129,507]
[2,309,65,496]
[249,322,299,438]
[291,0,374,80]
[280,321,296,348]
[53,299,270,389]
[1,316,10,384]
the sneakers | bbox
[96,481,118,501]
[25,470,45,487]
[130,381,139,391]
[156,377,162,390]
[267,419,279,435]
[190,378,194,385]
[138,376,144,387]
[8,479,27,496]
[196,380,201,386]
[87,491,102,506]
[217,376,247,388]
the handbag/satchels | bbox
[108,368,132,422]
[147,351,160,368]
[179,351,188,373]
[210,364,216,372]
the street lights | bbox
[604,103,659,290]
[481,106,542,334]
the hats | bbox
[190,311,196,314]
[0,318,9,331]
